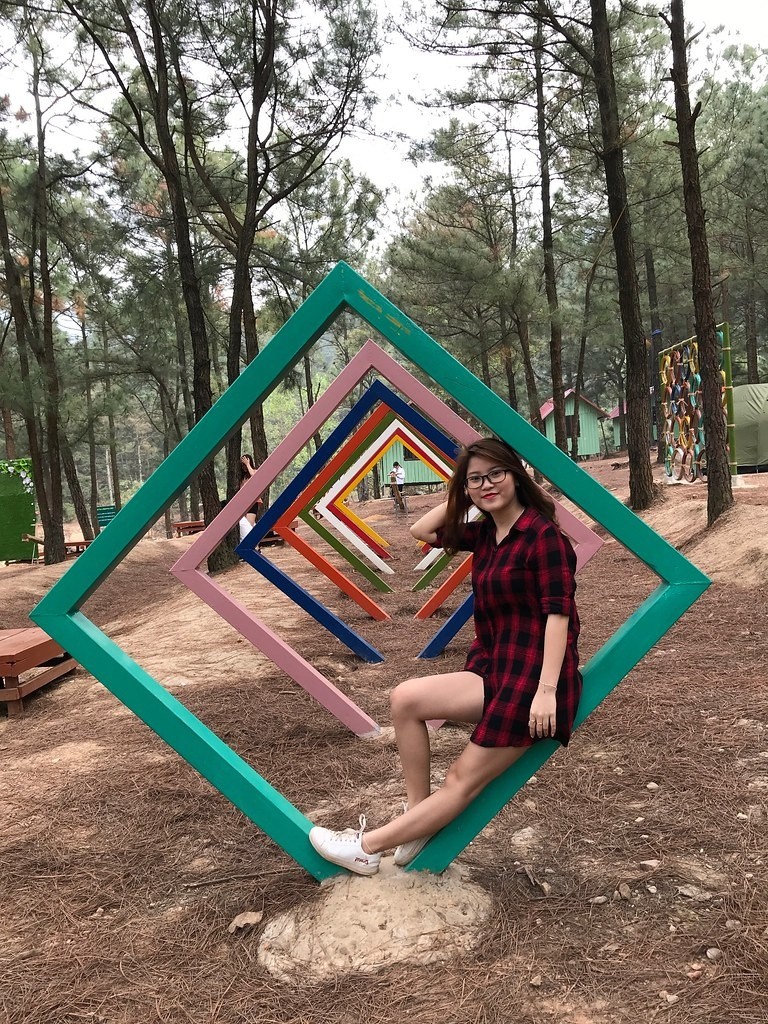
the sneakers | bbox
[309,813,382,876]
[394,800,434,865]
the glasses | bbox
[463,469,509,489]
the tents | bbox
[733,381,768,474]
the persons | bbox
[219,453,264,564]
[306,437,583,878]
[387,461,405,497]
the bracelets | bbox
[539,680,557,690]
